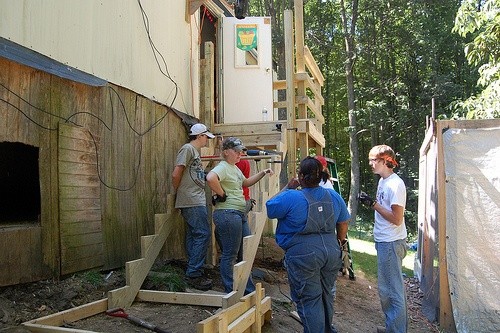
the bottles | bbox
[247,150,266,155]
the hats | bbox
[222,137,248,152]
[314,156,327,169]
[189,124,215,139]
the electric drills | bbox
[247,150,269,161]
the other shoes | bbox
[377,325,386,333]
[188,277,212,289]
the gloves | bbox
[210,193,226,205]
[358,193,377,207]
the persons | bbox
[358,145,409,333]
[206,137,274,295]
[266,156,351,333]
[172,123,217,291]
